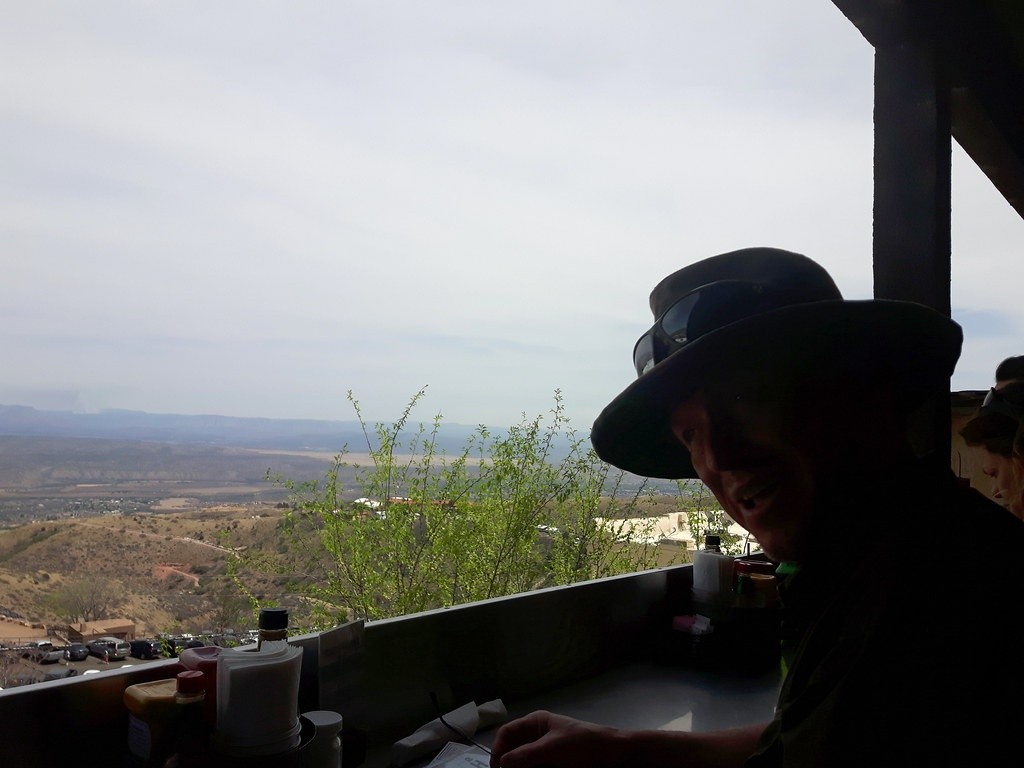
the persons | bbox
[101,650,111,666]
[959,354,1024,520]
[64,649,74,666]
[487,247,1024,768]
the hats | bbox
[590,247,963,479]
[959,356,1024,443]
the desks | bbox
[342,646,783,768]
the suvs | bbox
[85,636,132,662]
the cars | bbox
[164,629,258,658]
[128,638,163,661]
[63,642,90,662]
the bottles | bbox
[122,646,220,768]
[256,608,289,651]
[300,710,343,768]
[734,558,777,612]
[705,535,720,552]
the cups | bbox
[211,712,317,768]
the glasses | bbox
[633,279,768,380]
[980,387,1010,409]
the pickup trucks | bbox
[24,639,65,665]
